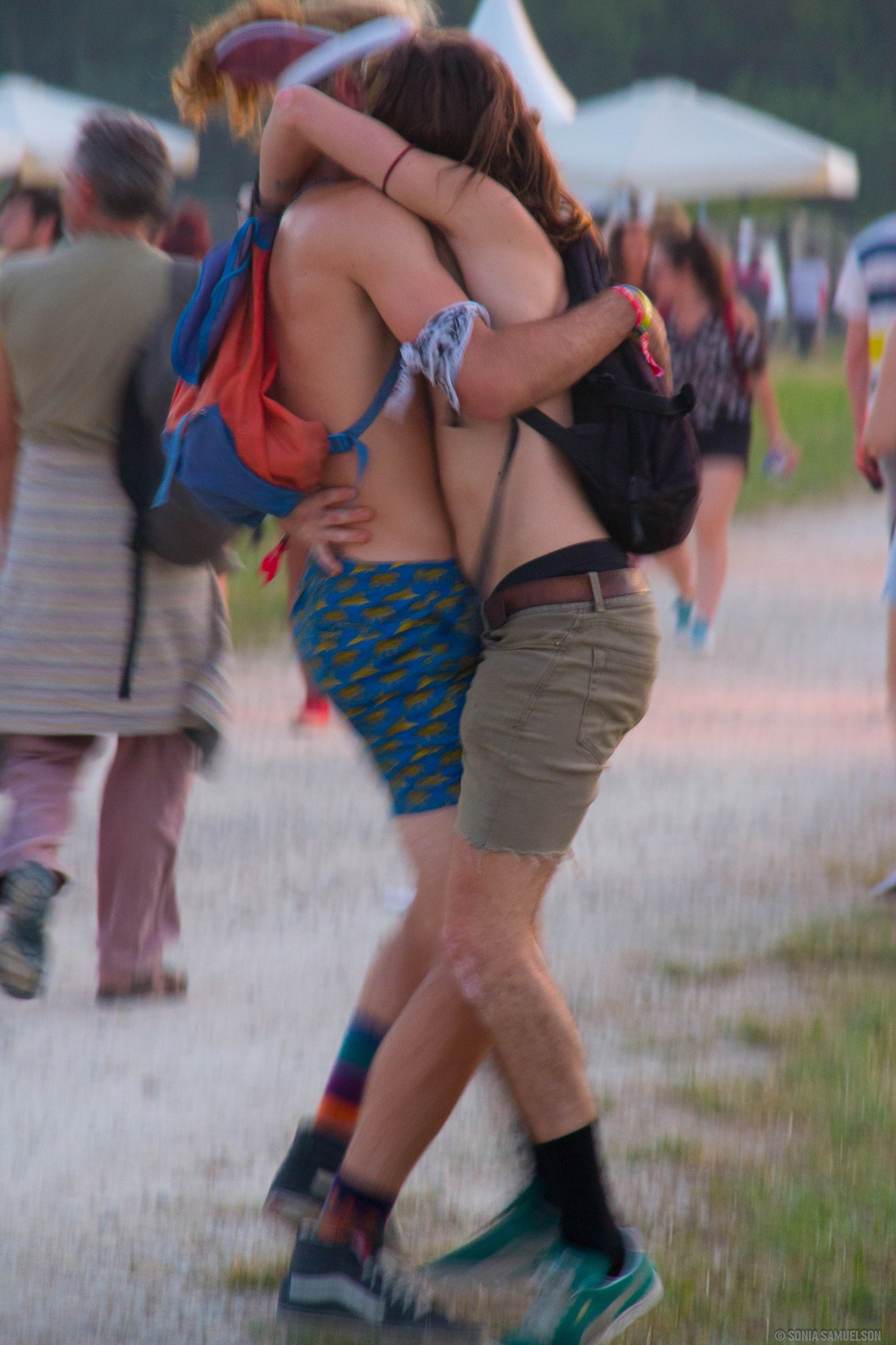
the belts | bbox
[493,568,648,615]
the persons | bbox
[249,23,669,1345]
[1,103,896,1003]
[168,2,489,1234]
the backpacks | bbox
[110,254,237,565]
[520,219,703,559]
[149,217,406,524]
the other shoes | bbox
[293,700,329,734]
[0,865,57,997]
[98,968,186,1003]
[673,597,709,650]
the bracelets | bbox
[612,282,668,378]
[379,144,416,193]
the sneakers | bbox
[260,1129,403,1256]
[495,1226,666,1345]
[277,1223,480,1345]
[413,1181,567,1329]
